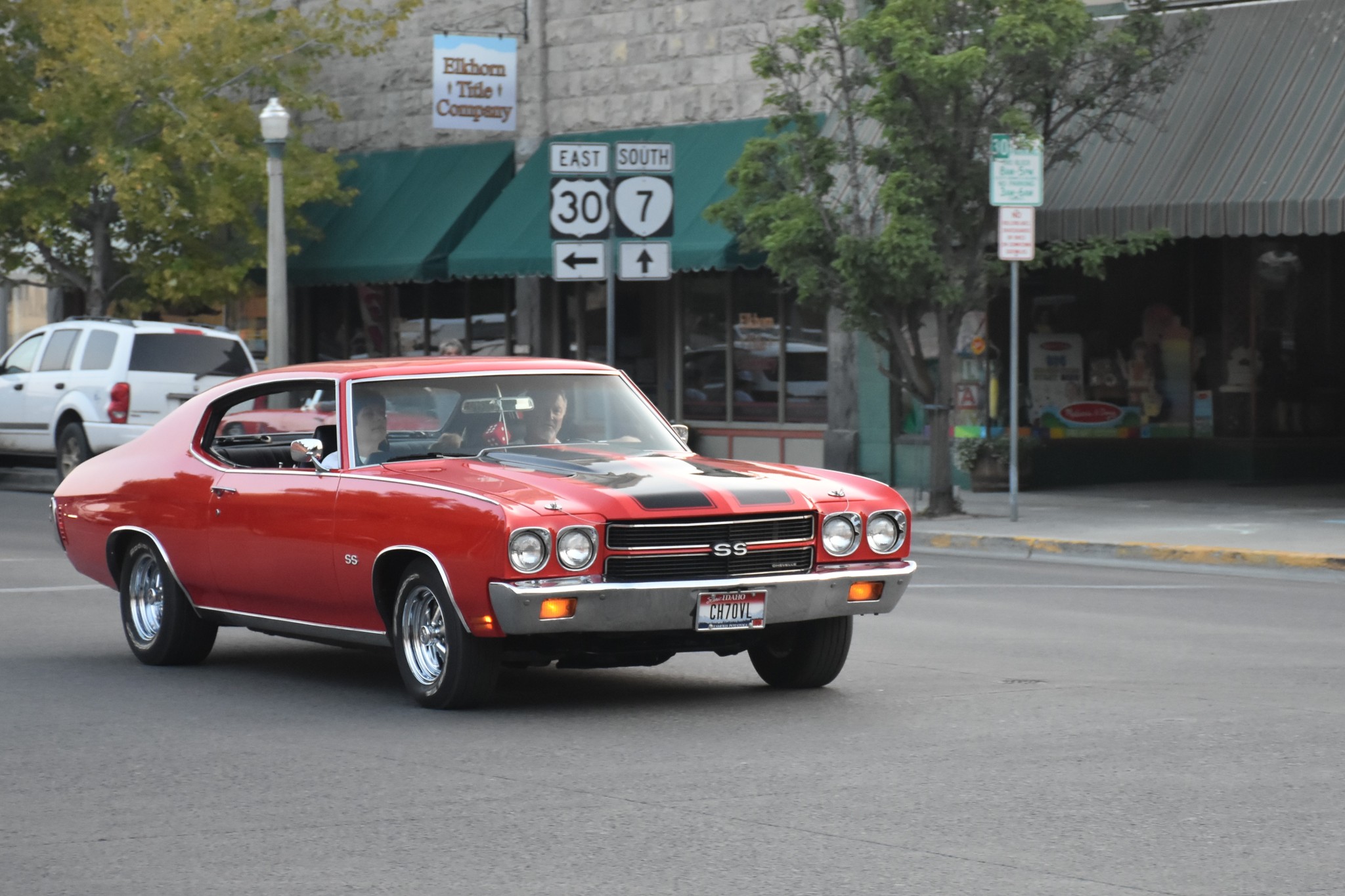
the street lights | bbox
[257,98,291,409]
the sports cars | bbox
[48,354,920,711]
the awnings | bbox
[245,140,517,287]
[448,111,828,277]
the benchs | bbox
[215,438,438,471]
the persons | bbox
[428,337,468,428]
[686,371,708,403]
[731,381,753,403]
[509,386,571,447]
[316,387,390,471]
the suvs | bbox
[0,312,267,486]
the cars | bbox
[681,323,831,410]
